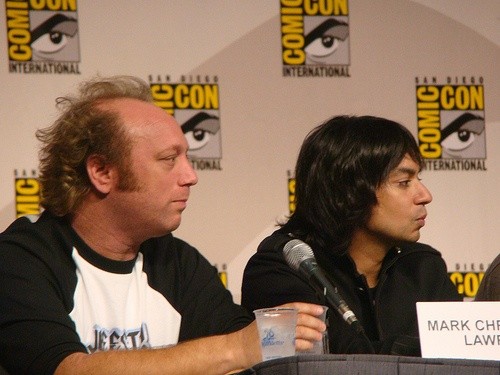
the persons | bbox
[0,76,326,375]
[239,114,463,360]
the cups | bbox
[301,303,329,354]
[253,307,301,361]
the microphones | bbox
[285,239,366,335]
